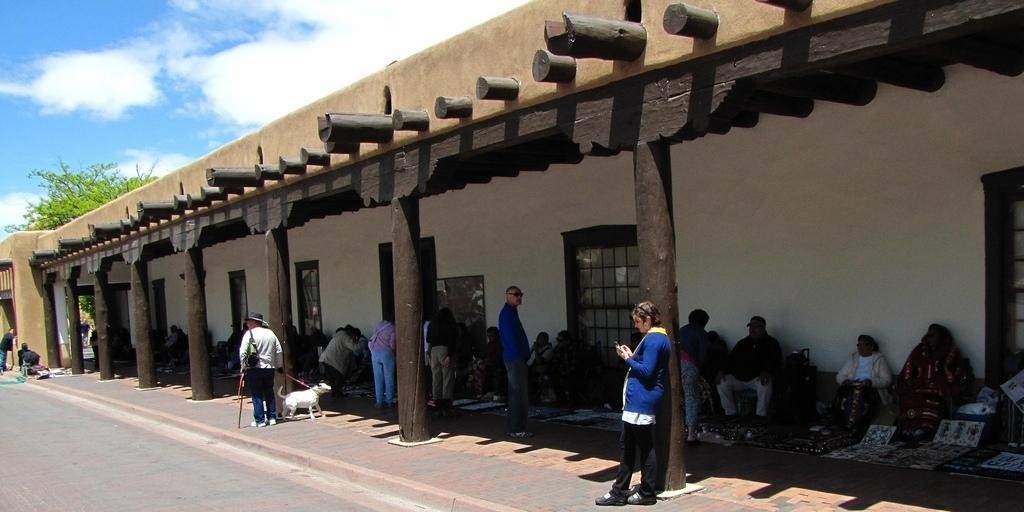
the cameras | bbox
[248,355,259,367]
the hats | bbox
[747,316,765,328]
[244,312,269,328]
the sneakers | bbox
[265,418,277,426]
[250,421,266,427]
[809,424,826,432]
[625,491,656,505]
[595,491,626,506]
[426,398,437,407]
[509,431,534,438]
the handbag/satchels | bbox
[244,329,259,367]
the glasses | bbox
[506,292,523,297]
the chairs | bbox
[993,349,1023,446]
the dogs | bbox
[277,382,332,422]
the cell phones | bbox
[613,340,620,349]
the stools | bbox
[735,388,762,417]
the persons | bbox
[896,324,975,436]
[836,335,893,425]
[715,316,781,422]
[707,331,729,417]
[595,300,672,506]
[671,310,713,446]
